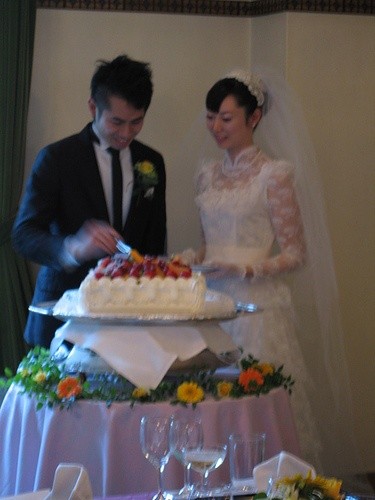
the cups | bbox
[228,431,266,488]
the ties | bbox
[89,122,123,237]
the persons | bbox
[10,54,167,351]
[175,72,373,495]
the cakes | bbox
[78,253,208,313]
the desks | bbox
[0,367,301,500]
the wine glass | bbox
[168,409,202,500]
[138,415,174,500]
[184,442,227,497]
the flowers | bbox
[275,468,347,500]
[136,160,159,200]
[0,344,296,412]
